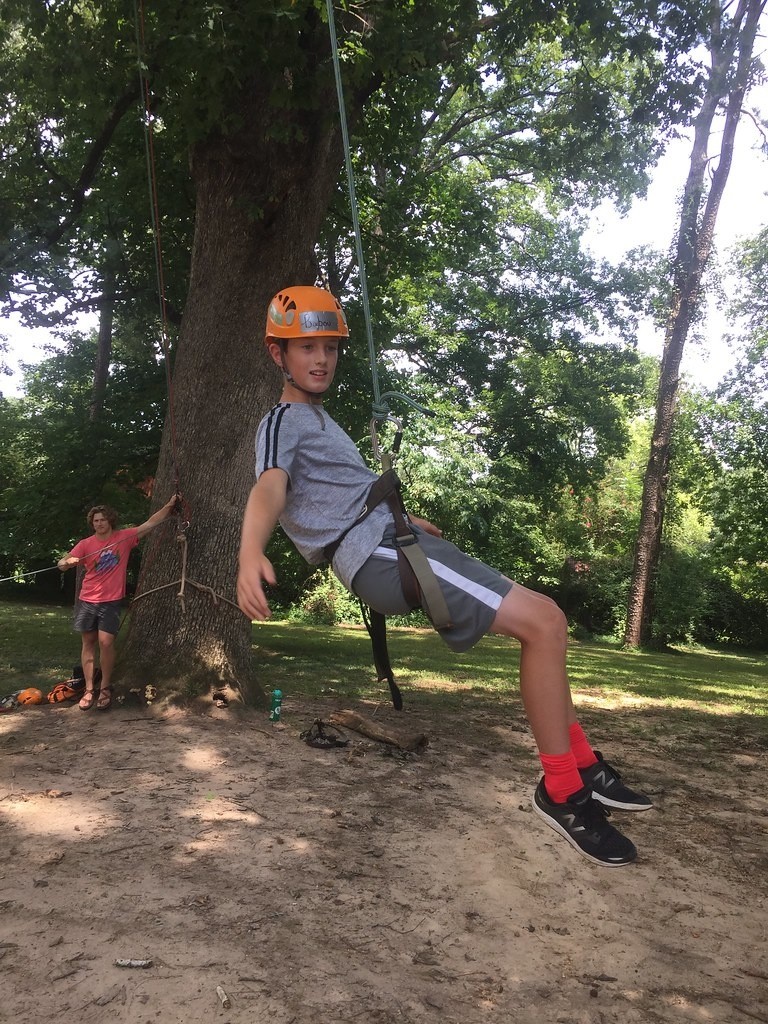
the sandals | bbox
[97,685,113,709]
[80,688,95,709]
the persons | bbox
[58,491,183,710]
[237,287,654,869]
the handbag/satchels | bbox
[0,689,24,711]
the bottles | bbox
[269,690,282,721]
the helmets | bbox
[266,286,350,343]
[17,688,42,706]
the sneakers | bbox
[532,775,638,868]
[584,750,654,811]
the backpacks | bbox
[47,665,98,703]
